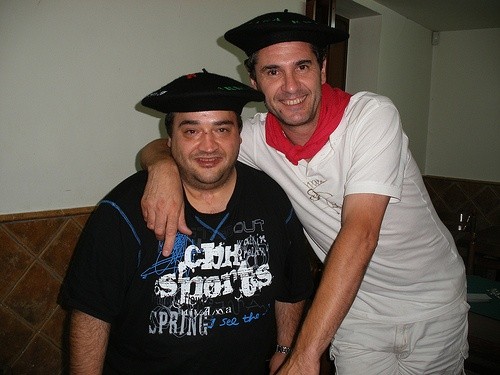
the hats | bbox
[141,69,266,113]
[225,9,350,57]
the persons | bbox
[56,69,324,375]
[137,7,470,375]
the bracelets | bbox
[274,344,294,355]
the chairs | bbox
[451,232,476,274]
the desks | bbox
[471,224,500,281]
